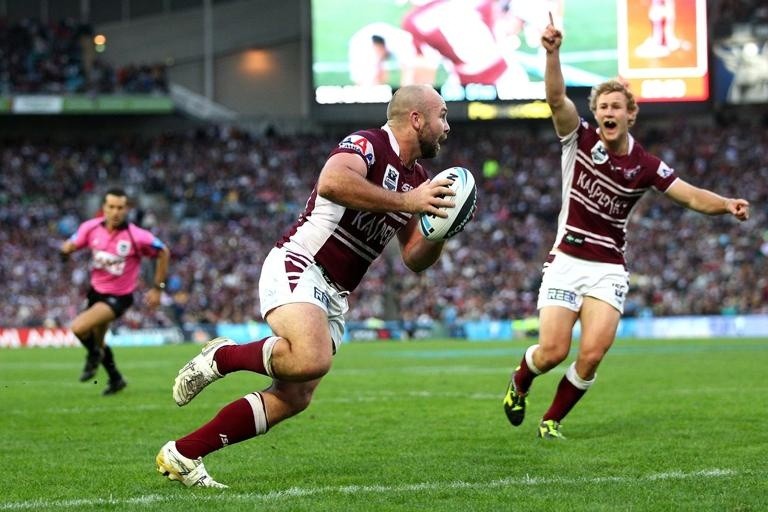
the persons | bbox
[58,187,171,395]
[502,9,751,440]
[156,84,479,488]
[0,2,767,343]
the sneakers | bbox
[537,418,568,439]
[503,366,530,426]
[171,336,237,407]
[102,376,128,395]
[156,439,230,488]
[79,357,97,381]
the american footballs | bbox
[418,166,476,239]
